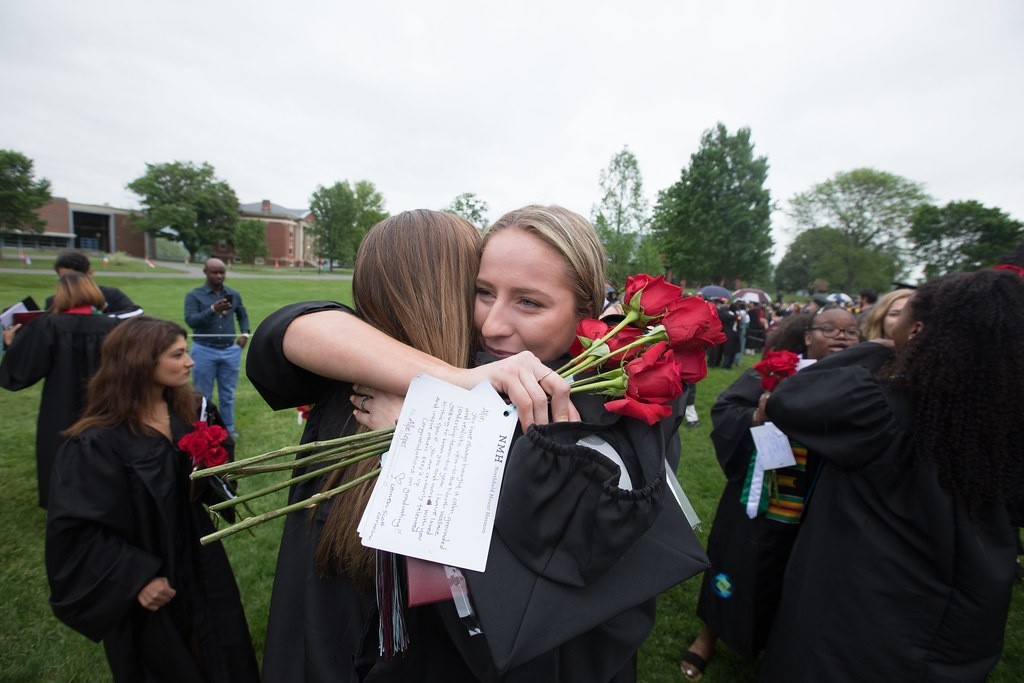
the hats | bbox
[54,250,91,275]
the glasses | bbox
[805,324,862,341]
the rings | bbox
[538,370,553,383]
[361,397,373,414]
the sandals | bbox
[680,640,717,682]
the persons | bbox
[245,204,694,683]
[45,250,144,323]
[44,315,260,683]
[681,242,1024,683]
[0,271,121,511]
[184,257,251,436]
[854,288,877,326]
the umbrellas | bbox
[700,286,731,298]
[732,288,772,303]
[826,293,852,302]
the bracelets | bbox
[211,304,216,312]
[242,333,248,337]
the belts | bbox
[193,338,234,350]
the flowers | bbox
[177,420,228,467]
[298,403,315,421]
[751,349,800,506]
[191,271,727,546]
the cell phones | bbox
[224,295,233,304]
